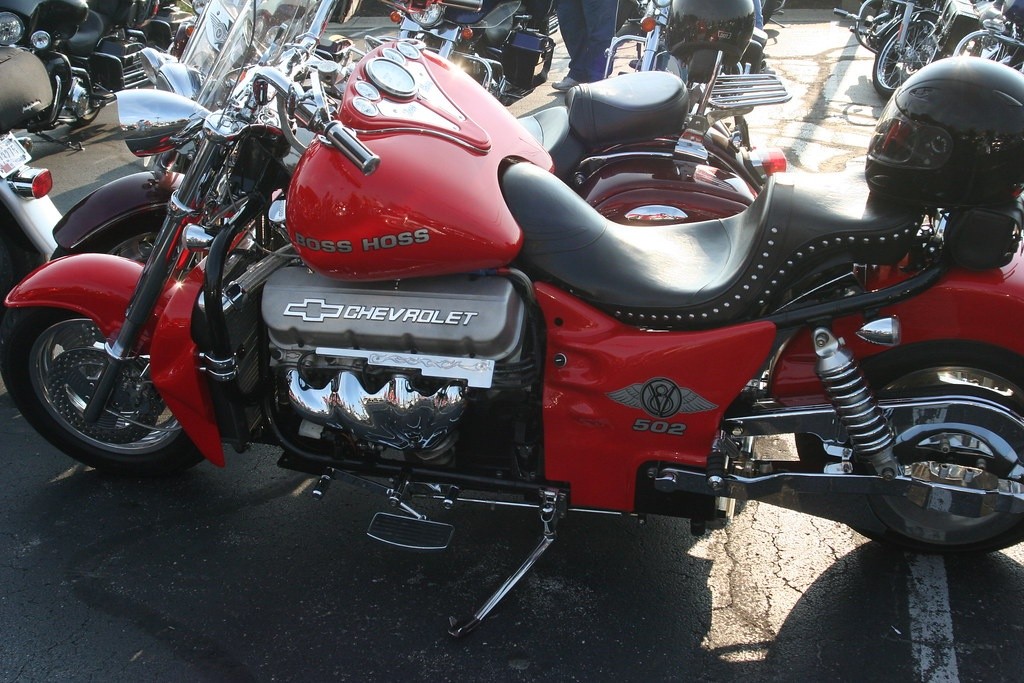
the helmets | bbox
[865,55,1024,210]
[665,0,756,67]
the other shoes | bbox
[630,60,643,70]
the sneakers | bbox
[552,77,580,91]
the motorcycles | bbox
[1,0,1024,638]
[1,0,1024,297]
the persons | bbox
[551,0,619,91]
